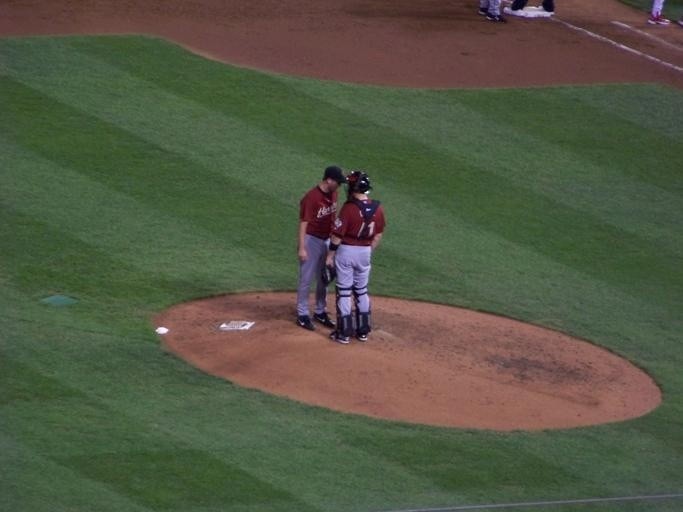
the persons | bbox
[647,0,682,27]
[295,164,346,331]
[323,169,386,345]
[477,0,507,23]
[501,0,557,19]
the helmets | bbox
[344,170,372,192]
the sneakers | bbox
[478,7,488,15]
[485,13,504,22]
[647,14,670,25]
[297,313,368,343]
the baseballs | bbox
[156,327,168,334]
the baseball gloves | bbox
[321,266,336,284]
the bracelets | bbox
[327,241,339,251]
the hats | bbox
[324,166,346,183]
